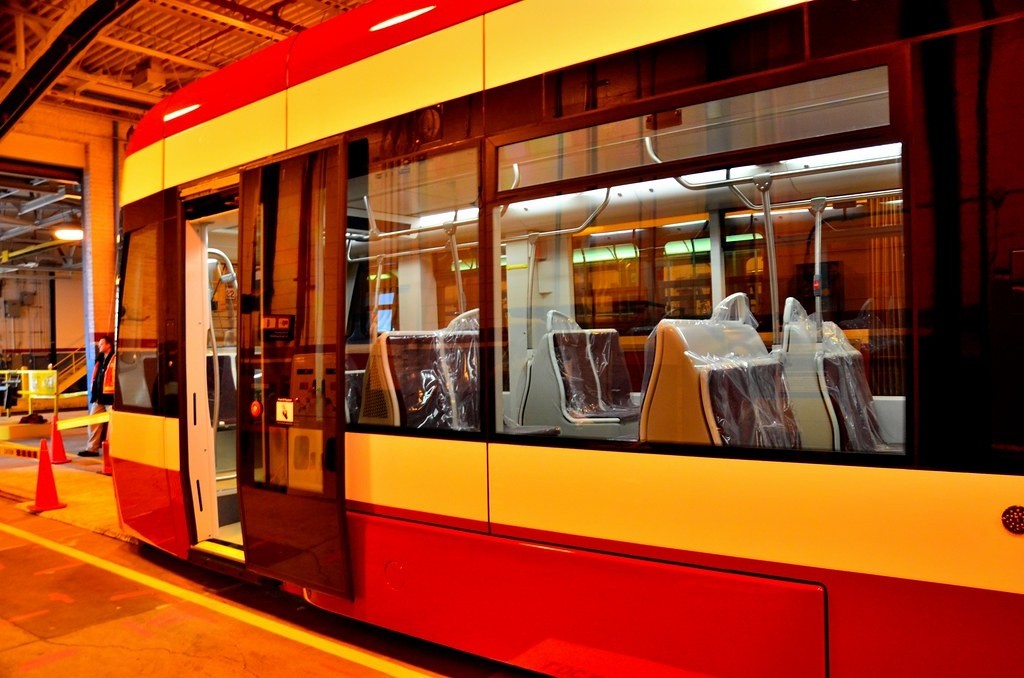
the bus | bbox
[108,0,1022,678]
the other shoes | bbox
[78,451,99,457]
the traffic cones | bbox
[49,415,72,465]
[26,438,68,513]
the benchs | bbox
[380,330,562,435]
[549,327,640,422]
[608,319,743,442]
[346,351,370,421]
[814,348,904,453]
[700,359,803,449]
[206,352,241,426]
[711,293,797,353]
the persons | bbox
[78,336,116,457]
